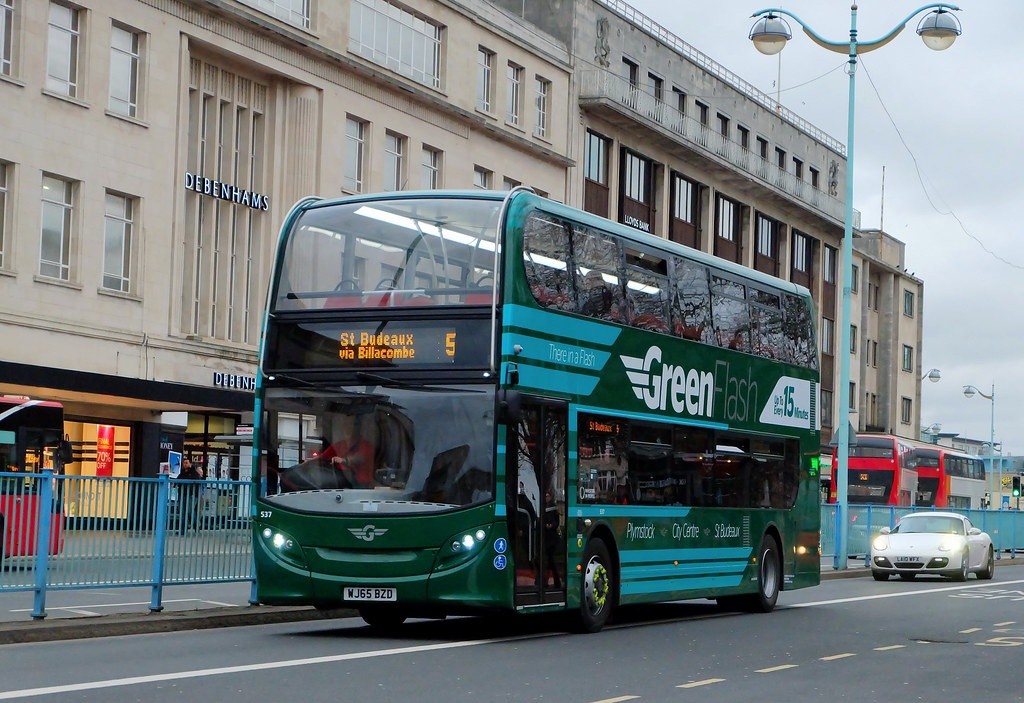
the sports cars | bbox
[870,511,995,582]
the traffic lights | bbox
[1012,476,1021,498]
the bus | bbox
[914,446,986,509]
[1,392,73,571]
[826,433,919,504]
[248,185,822,636]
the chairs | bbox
[322,279,773,358]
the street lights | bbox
[962,380,995,509]
[748,1,963,572]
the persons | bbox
[758,442,801,509]
[535,490,561,588]
[171,459,204,536]
[305,414,382,489]
